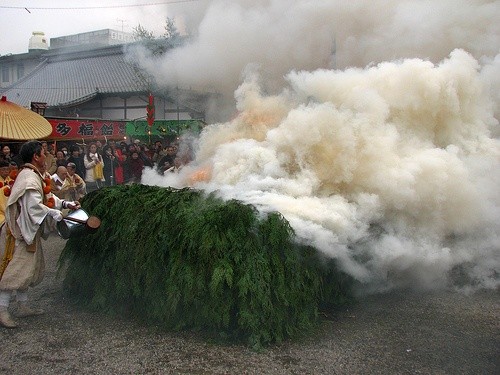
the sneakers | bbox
[0,305,17,328]
[15,304,45,317]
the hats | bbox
[0,160,9,168]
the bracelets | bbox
[62,200,67,209]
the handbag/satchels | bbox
[93,162,103,181]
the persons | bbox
[0,160,13,224]
[39,161,52,182]
[0,140,88,178]
[50,165,75,202]
[83,141,104,194]
[96,138,196,186]
[65,163,87,202]
[9,162,18,173]
[0,139,81,328]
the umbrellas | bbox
[0,94,53,140]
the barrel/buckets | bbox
[56,208,92,239]
